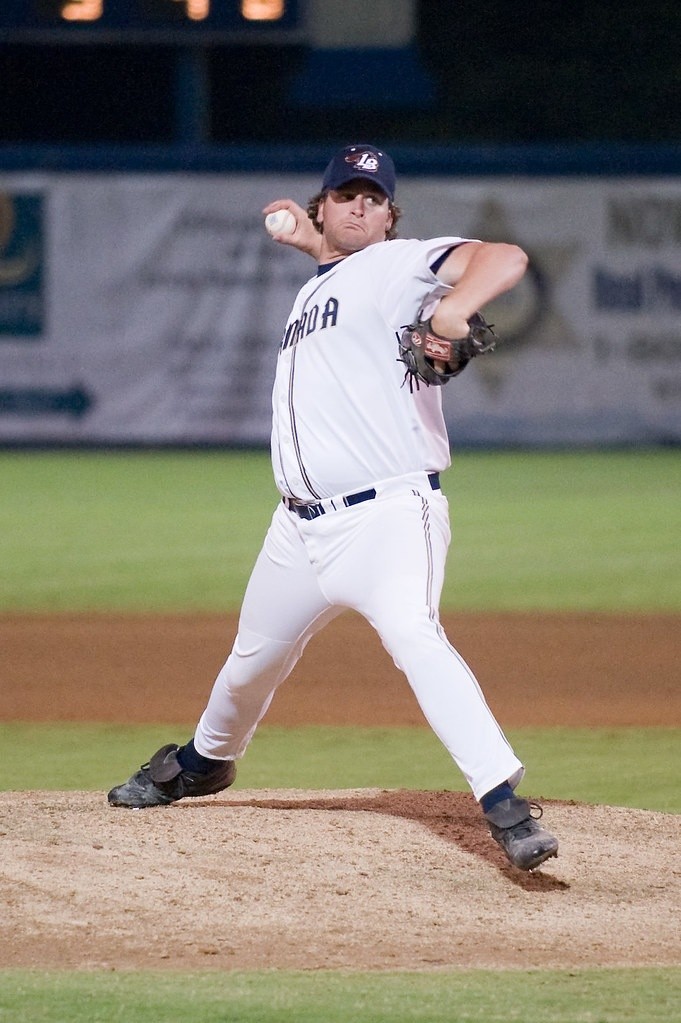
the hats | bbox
[322,144,397,202]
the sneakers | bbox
[485,795,560,874]
[106,742,238,809]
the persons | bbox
[108,144,558,869]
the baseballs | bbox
[264,209,297,237]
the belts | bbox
[280,472,441,521]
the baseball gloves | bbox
[395,290,501,394]
